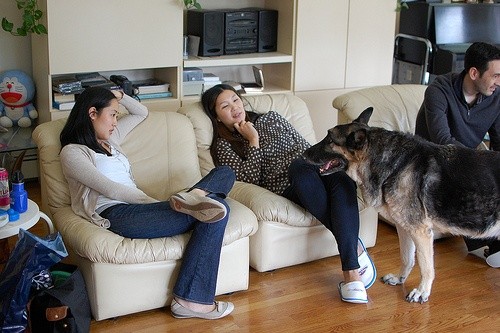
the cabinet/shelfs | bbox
[32,0,399,124]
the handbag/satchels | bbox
[30,262,91,333]
[0,228,68,333]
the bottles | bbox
[9,172,27,213]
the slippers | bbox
[486,250,500,268]
[338,281,368,304]
[467,245,489,260]
[357,237,376,289]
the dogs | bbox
[301,107,500,305]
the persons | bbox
[415,42,500,268]
[201,83,377,303]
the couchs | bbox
[175,94,379,276]
[32,112,259,321]
[332,84,489,240]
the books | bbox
[53,72,124,111]
[131,78,174,100]
[60,87,236,320]
[183,68,222,96]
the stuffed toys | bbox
[0,69,38,128]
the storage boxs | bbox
[183,80,205,96]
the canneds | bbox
[0,168,10,206]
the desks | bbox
[0,197,54,268]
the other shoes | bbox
[170,297,234,319]
[169,191,227,223]
